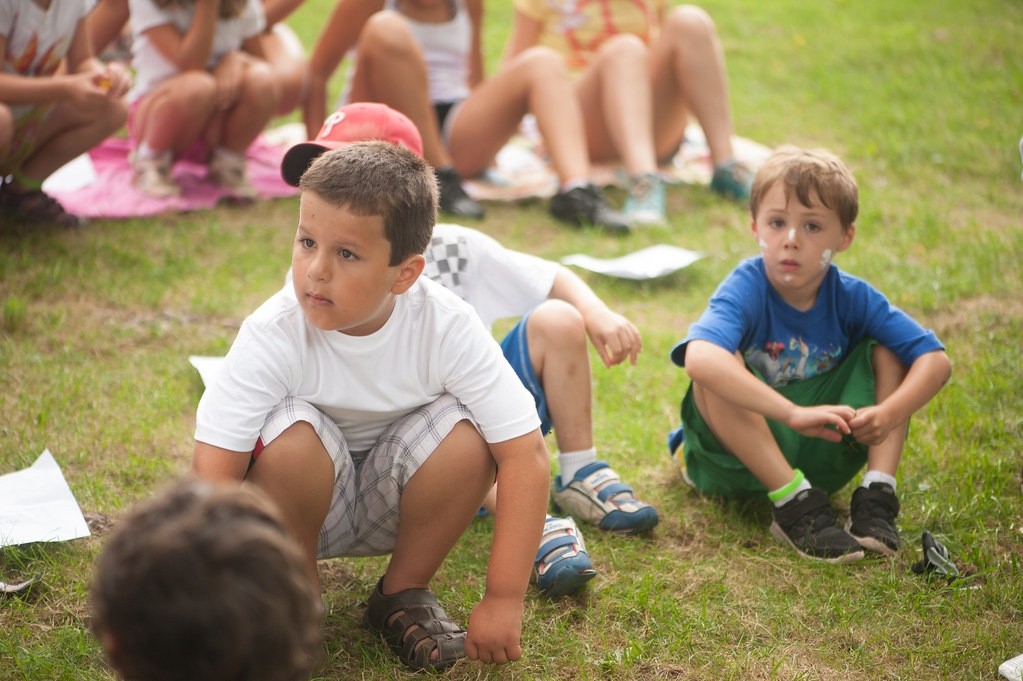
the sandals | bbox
[363,573,469,675]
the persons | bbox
[0,0,773,234]
[83,476,324,680]
[667,146,953,563]
[281,103,658,595]
[189,142,552,675]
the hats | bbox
[281,100,425,187]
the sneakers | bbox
[768,487,865,565]
[532,511,597,597]
[552,461,659,535]
[843,480,900,555]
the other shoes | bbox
[548,183,631,238]
[709,158,755,211]
[206,155,256,207]
[126,149,181,198]
[0,174,78,228]
[620,172,671,228]
[435,164,485,219]
